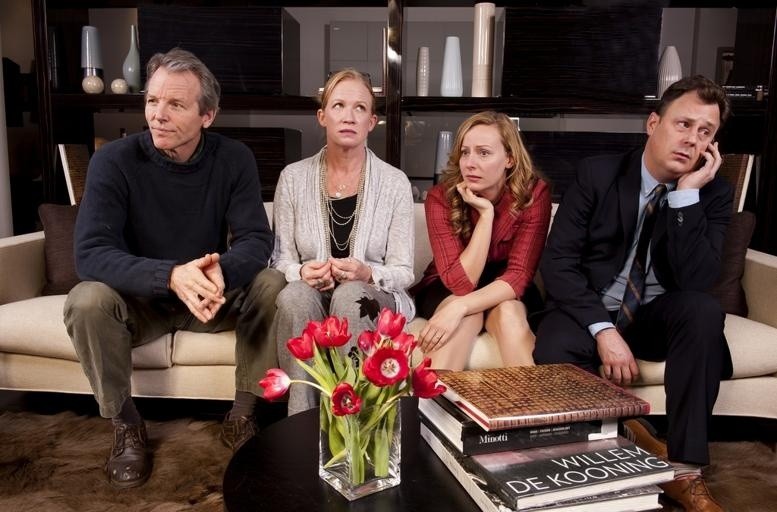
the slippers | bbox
[623,415,668,460]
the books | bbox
[419,421,675,512]
[426,364,650,456]
[56,143,89,205]
[719,154,755,214]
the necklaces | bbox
[317,144,368,259]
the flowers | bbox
[257,307,449,481]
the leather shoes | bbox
[216,411,260,453]
[103,420,154,489]
[658,473,728,512]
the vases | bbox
[318,393,401,498]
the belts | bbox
[614,183,669,336]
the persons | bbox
[270,69,416,415]
[63,51,287,485]
[405,112,552,372]
[540,77,734,510]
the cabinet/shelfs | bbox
[392,0,767,242]
[33,3,396,186]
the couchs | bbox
[1,198,770,421]
